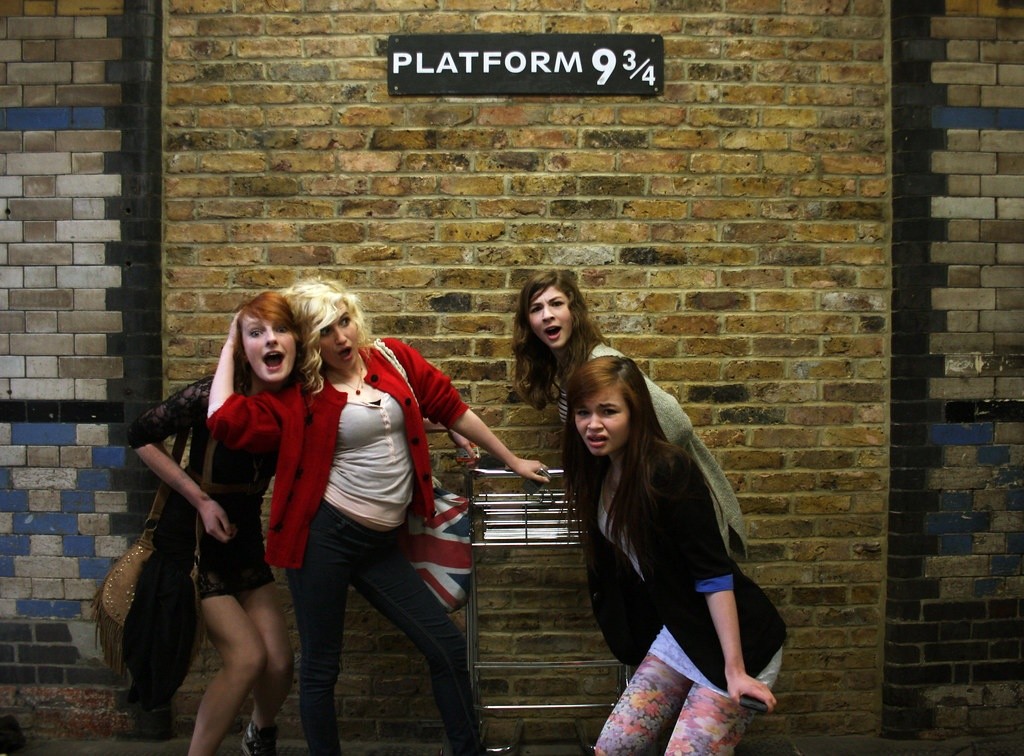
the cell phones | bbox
[739,695,768,712]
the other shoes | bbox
[240,721,278,756]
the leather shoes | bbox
[477,743,518,756]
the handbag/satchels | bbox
[93,537,208,680]
[401,477,474,612]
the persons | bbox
[513,269,788,756]
[128,290,302,756]
[206,276,549,756]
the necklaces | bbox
[322,355,363,395]
